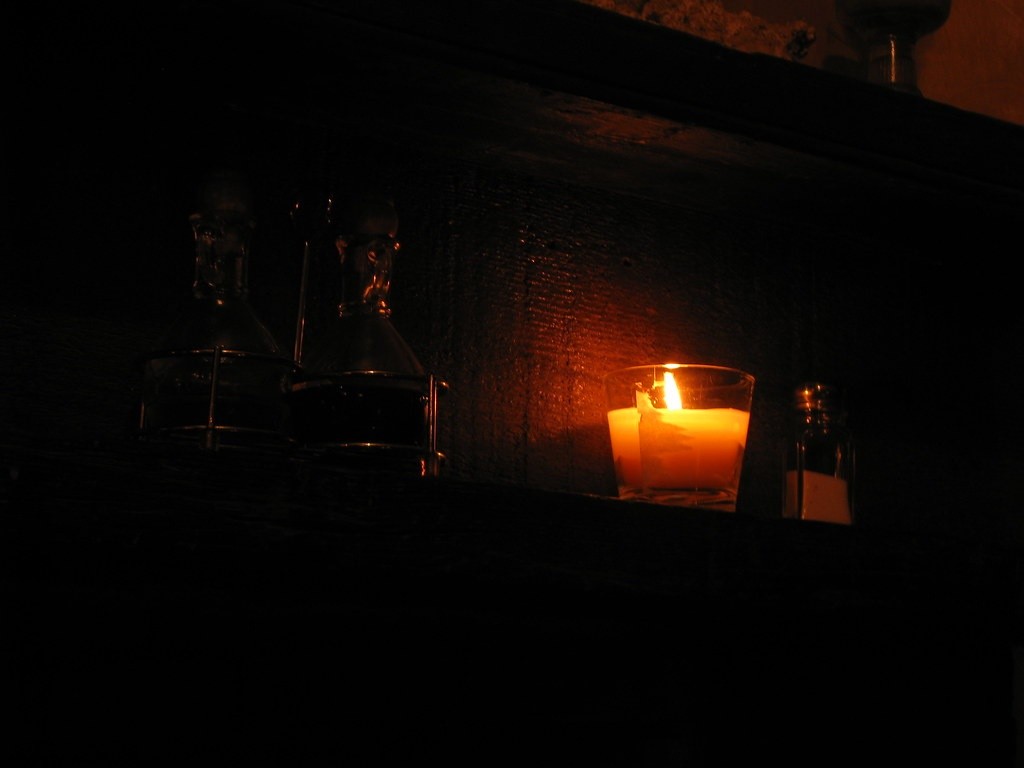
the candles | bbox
[608,371,751,491]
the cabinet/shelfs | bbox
[0,1,1024,768]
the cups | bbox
[603,364,755,511]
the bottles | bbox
[782,381,854,525]
[139,167,285,452]
[288,185,430,460]
